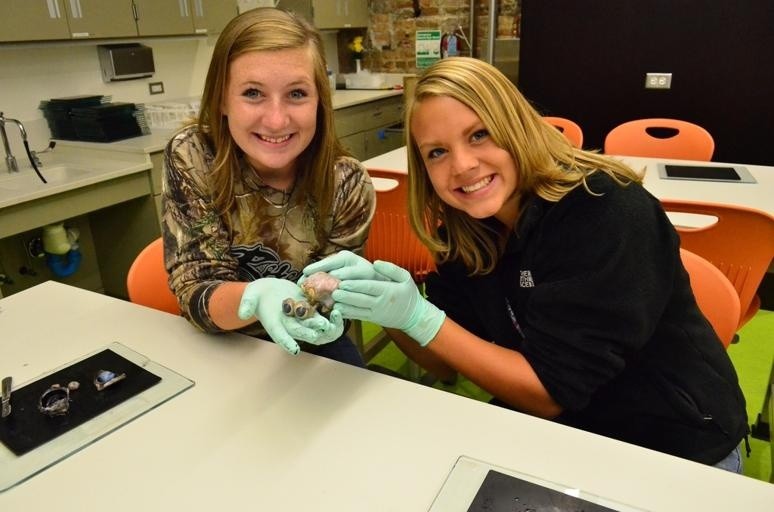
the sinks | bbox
[0,165,98,203]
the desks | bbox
[349,145,772,369]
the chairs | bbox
[676,246,743,353]
[602,118,718,163]
[541,117,586,152]
[357,165,439,283]
[128,234,185,317]
[658,194,772,335]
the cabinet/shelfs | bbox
[331,94,406,164]
[85,146,174,301]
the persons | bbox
[297,55,753,473]
[157,5,376,371]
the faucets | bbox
[1,112,27,172]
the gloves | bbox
[332,260,447,348]
[296,250,393,287]
[237,277,345,357]
